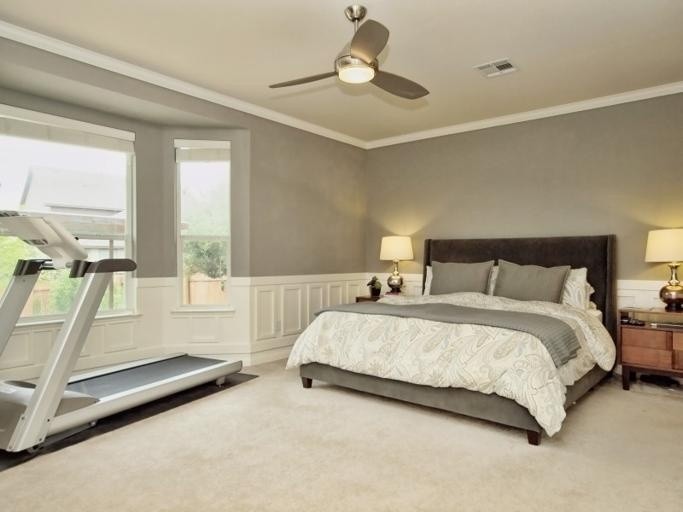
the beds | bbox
[296,234,614,448]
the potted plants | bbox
[367,277,380,296]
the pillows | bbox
[423,258,595,314]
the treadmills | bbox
[0,211,242,455]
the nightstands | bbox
[615,309,683,393]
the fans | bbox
[264,7,431,102]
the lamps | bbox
[333,53,379,85]
[379,237,415,293]
[643,228,682,312]
